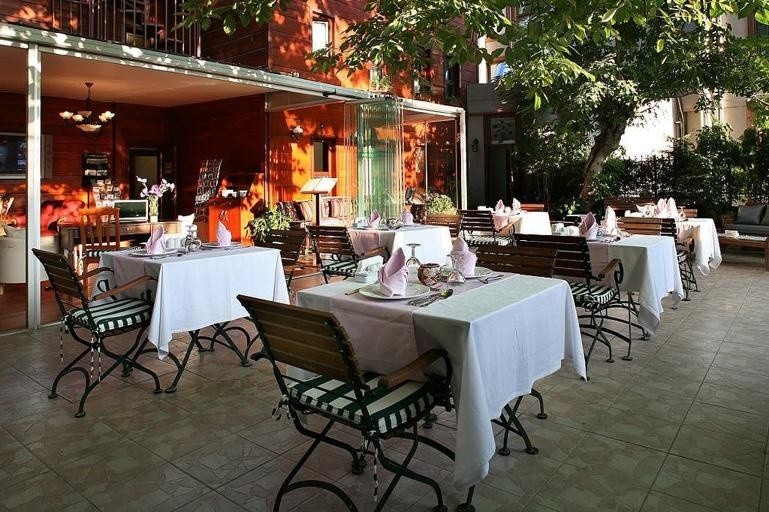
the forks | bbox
[477,274,504,284]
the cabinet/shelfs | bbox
[81,152,112,188]
[208,172,264,246]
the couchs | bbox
[1,181,95,234]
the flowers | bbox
[133,175,177,216]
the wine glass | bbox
[609,216,623,241]
[678,205,687,222]
[405,243,422,283]
[445,254,467,294]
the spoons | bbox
[419,289,453,307]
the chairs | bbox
[0,224,74,295]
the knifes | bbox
[407,293,442,306]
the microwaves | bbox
[110,200,148,224]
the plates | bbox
[442,267,494,279]
[358,283,430,300]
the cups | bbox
[352,210,414,230]
[551,223,605,241]
[477,206,527,216]
[418,263,442,285]
[131,232,241,259]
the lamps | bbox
[471,138,478,153]
[288,124,304,143]
[58,82,115,136]
